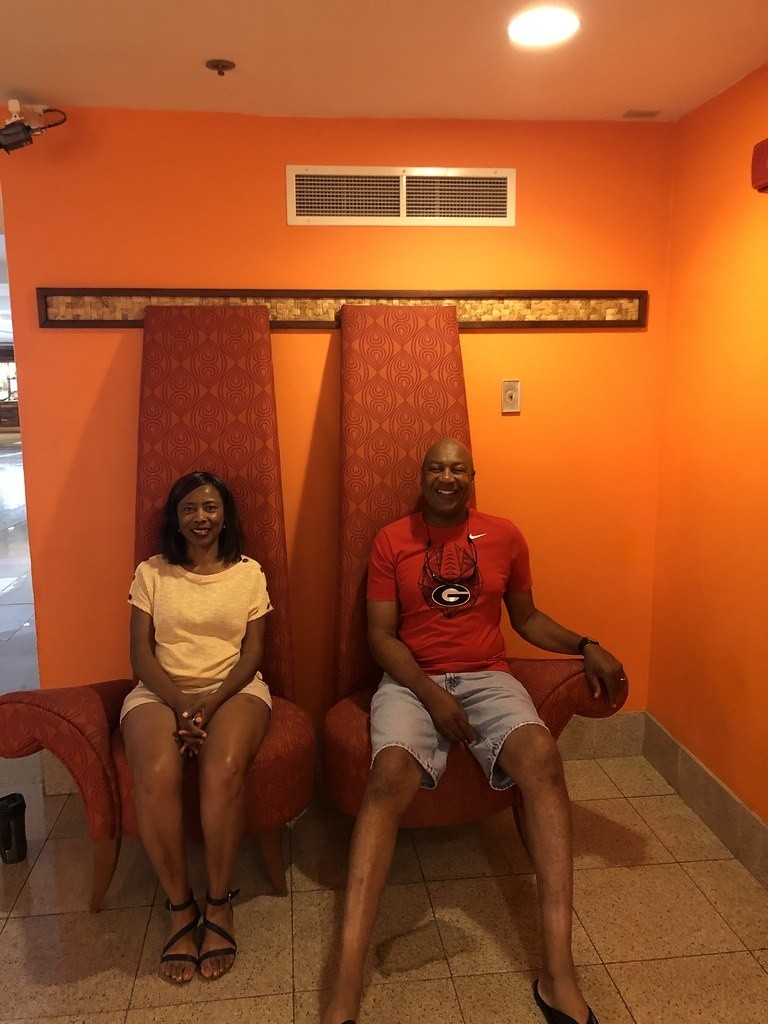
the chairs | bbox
[323,304,626,859]
[0,307,320,913]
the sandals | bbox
[161,889,200,982]
[198,888,240,979]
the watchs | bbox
[578,637,600,654]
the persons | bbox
[323,439,626,1024]
[119,470,275,983]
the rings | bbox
[621,678,626,680]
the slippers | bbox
[533,978,598,1024]
